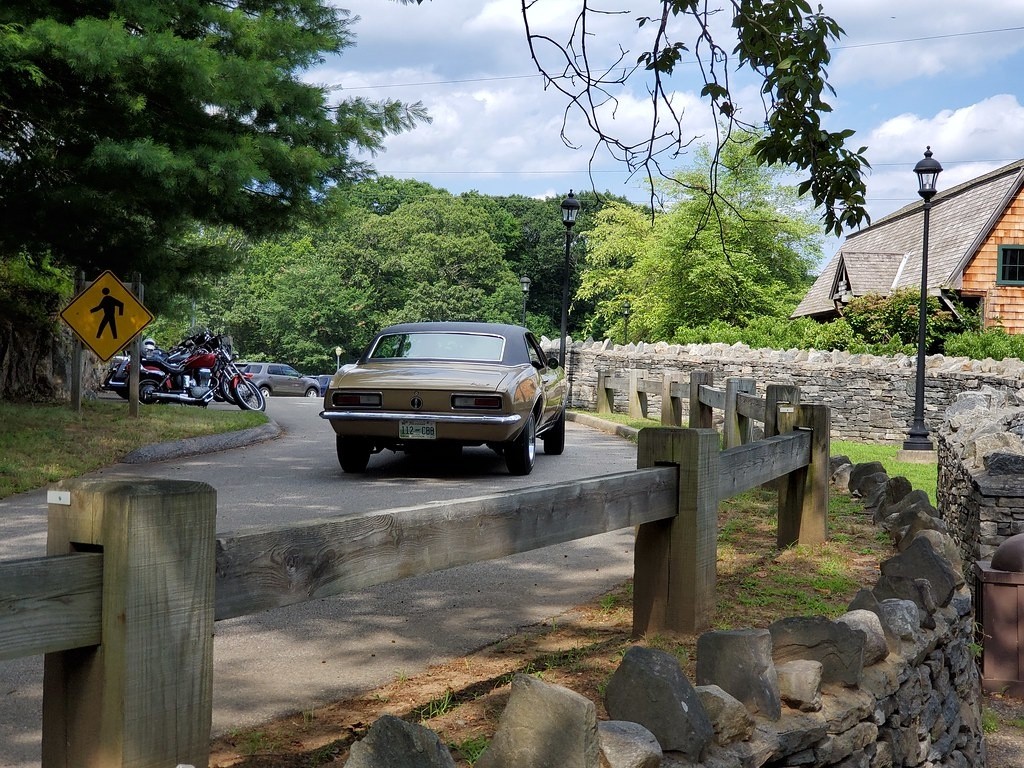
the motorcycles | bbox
[97,327,266,412]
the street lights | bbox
[559,189,581,369]
[622,298,630,345]
[902,145,943,450]
[520,272,530,328]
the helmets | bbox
[142,338,156,351]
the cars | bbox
[319,321,571,475]
[242,363,321,398]
